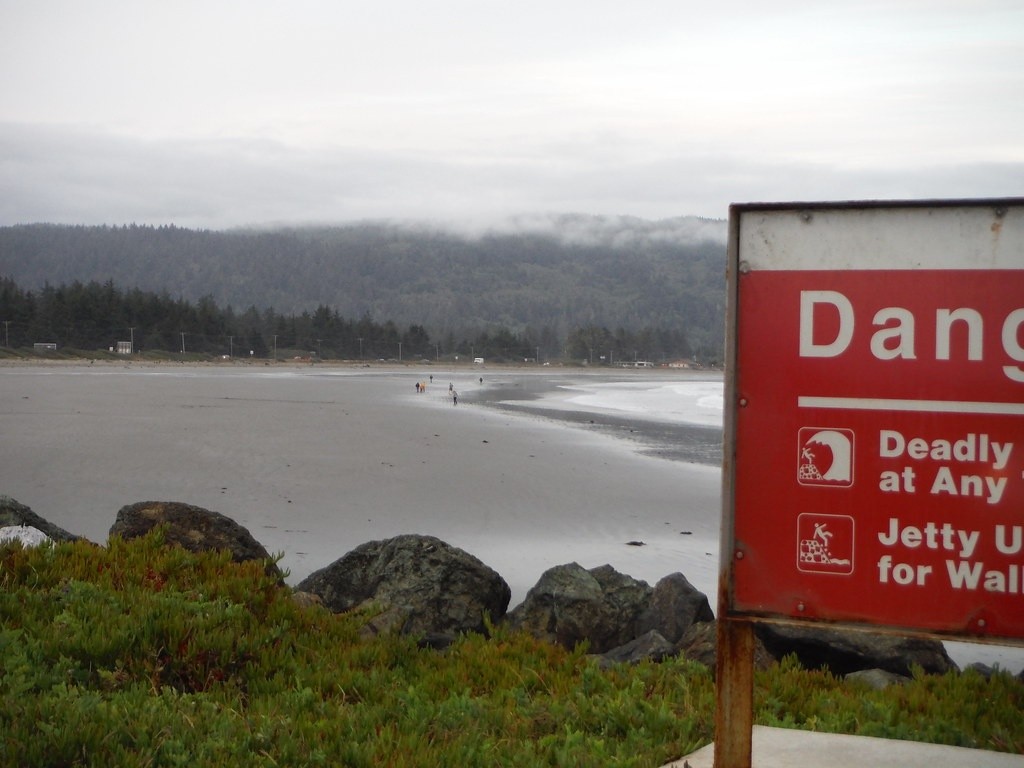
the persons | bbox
[479,377,483,383]
[430,375,433,382]
[416,382,420,392]
[449,383,453,394]
[420,383,425,392]
[453,391,458,405]
